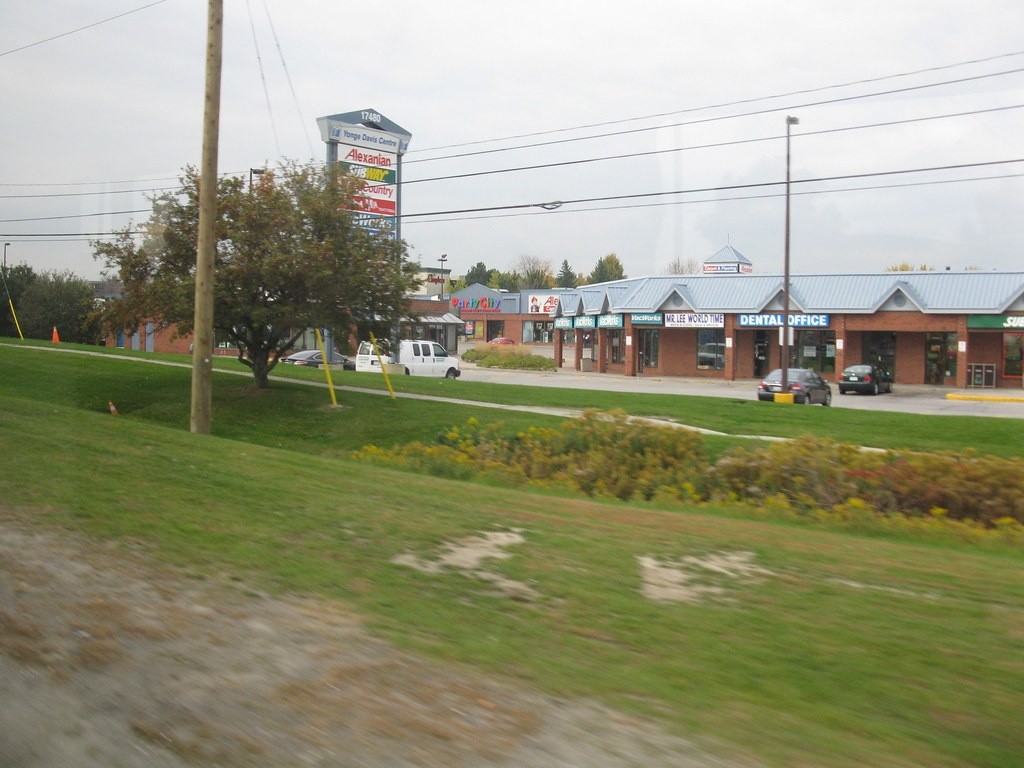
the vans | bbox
[355,337,461,379]
[698,342,727,366]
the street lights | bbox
[774,116,802,404]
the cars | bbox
[837,364,890,396]
[756,369,830,407]
[280,351,354,373]
[488,337,516,346]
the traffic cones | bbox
[50,325,60,344]
[108,400,118,414]
